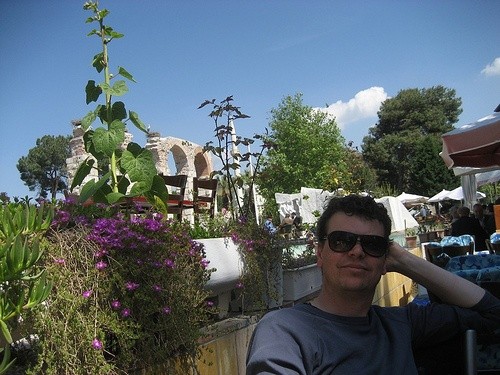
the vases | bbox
[282,262,323,301]
[263,260,283,309]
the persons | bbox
[419,197,500,252]
[265,211,302,233]
[246,194,500,375]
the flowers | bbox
[221,205,320,319]
[14,194,229,375]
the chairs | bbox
[121,172,218,228]
[274,224,292,238]
[411,232,500,375]
[291,217,302,238]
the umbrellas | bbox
[396,113,500,204]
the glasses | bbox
[322,230,388,257]
